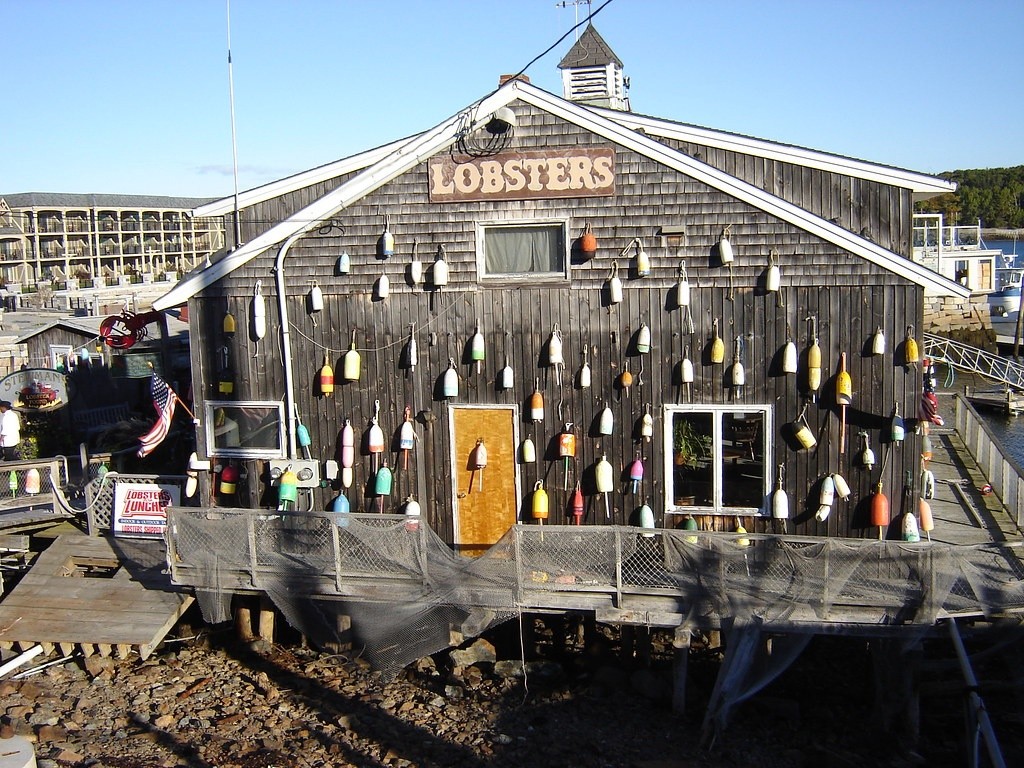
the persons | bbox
[0,401,20,461]
[80,344,92,375]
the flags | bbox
[922,358,944,425]
[137,362,176,458]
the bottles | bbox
[340,233,453,298]
[253,287,266,339]
[344,322,513,398]
[370,424,385,453]
[400,418,413,450]
[476,438,488,466]
[98,462,108,484]
[220,462,237,496]
[334,489,349,526]
[312,286,323,310]
[10,471,17,490]
[25,468,40,493]
[525,223,933,547]
[376,463,392,496]
[406,501,420,531]
[218,367,232,401]
[280,472,296,503]
[321,364,334,393]
[342,419,354,488]
[298,425,311,447]
[224,311,234,335]
